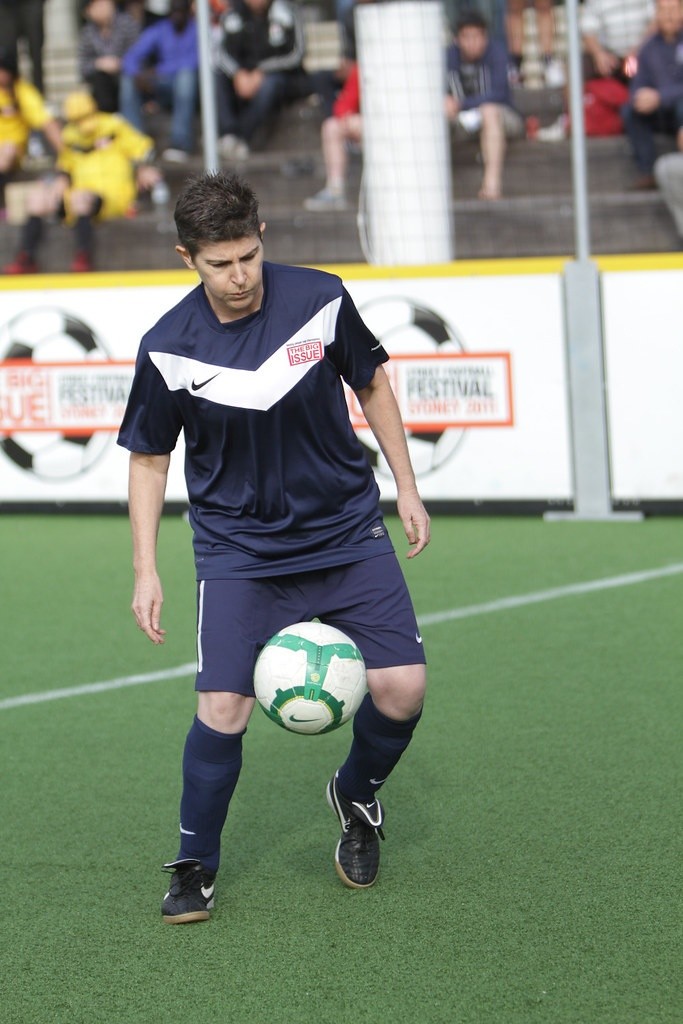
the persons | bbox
[0,0,308,275]
[117,172,432,925]
[303,0,525,211]
[579,0,683,247]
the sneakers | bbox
[160,859,217,924]
[325,770,385,888]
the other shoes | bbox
[533,124,566,143]
[71,253,92,272]
[4,252,36,275]
[162,148,192,163]
[478,183,500,201]
[217,134,248,159]
[303,191,347,211]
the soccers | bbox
[254,621,366,737]
